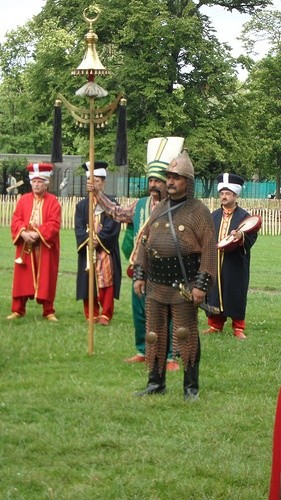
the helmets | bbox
[160,148,195,208]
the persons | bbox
[86,137,185,370]
[59,177,68,197]
[74,161,122,325]
[132,150,219,402]
[6,162,62,322]
[202,173,258,340]
[5,169,27,201]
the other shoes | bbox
[165,362,179,370]
[123,355,145,362]
[6,312,21,319]
[47,313,58,321]
[234,328,246,339]
[184,388,199,400]
[88,316,109,325]
[137,382,166,394]
[203,327,221,334]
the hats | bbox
[27,162,53,179]
[216,172,244,196]
[146,137,185,190]
[81,161,108,178]
